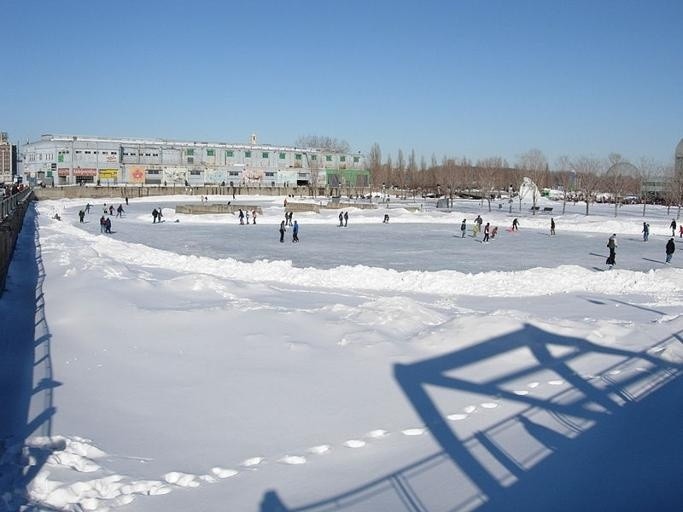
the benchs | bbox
[529,206,540,211]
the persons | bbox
[645,224,649,241]
[641,222,648,241]
[472,221,478,237]
[343,211,348,227]
[382,214,389,224]
[511,217,519,231]
[338,211,343,227]
[482,222,489,242]
[0,180,48,207]
[473,215,482,232]
[678,225,683,238]
[604,252,615,270]
[460,219,465,239]
[669,217,676,236]
[605,233,617,255]
[664,237,675,263]
[78,194,299,243]
[490,226,498,240]
[550,218,556,236]
